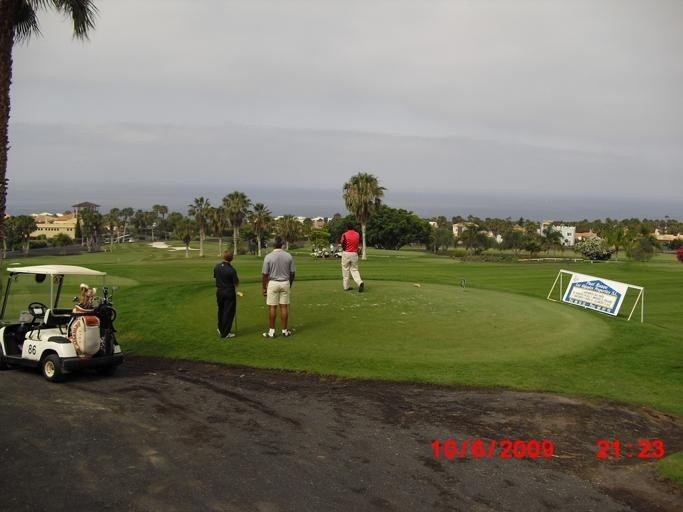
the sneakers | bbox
[226,333,236,338]
[358,282,364,291]
[263,332,275,338]
[348,286,353,290]
[282,330,292,336]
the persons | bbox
[212,251,239,339]
[259,235,296,338]
[339,222,365,292]
[311,243,335,258]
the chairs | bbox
[25,308,73,341]
